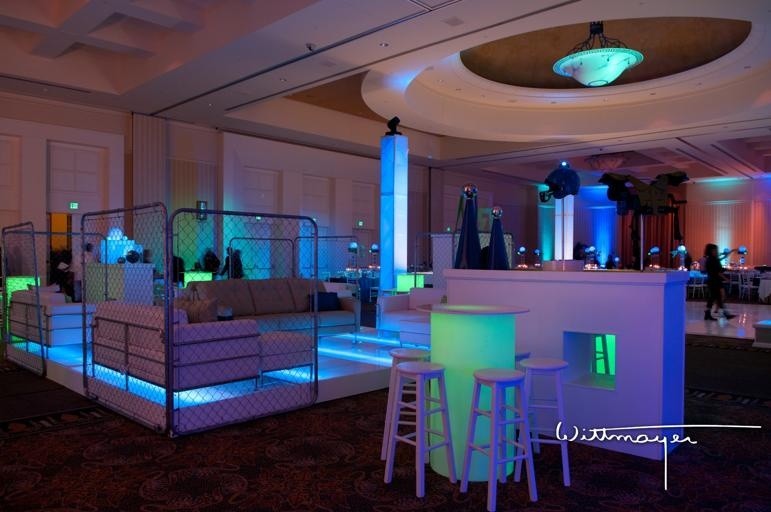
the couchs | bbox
[376,287,447,335]
[9,277,362,392]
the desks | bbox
[414,304,531,481]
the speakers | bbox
[722,312,737,319]
[704,310,717,321]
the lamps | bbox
[552,20,645,87]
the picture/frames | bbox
[414,304,531,481]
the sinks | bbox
[376,287,447,335]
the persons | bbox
[605,254,617,269]
[50,240,245,303]
[702,244,739,322]
[700,243,728,314]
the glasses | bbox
[552,20,645,87]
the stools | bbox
[381,347,572,512]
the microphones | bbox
[381,347,572,512]
